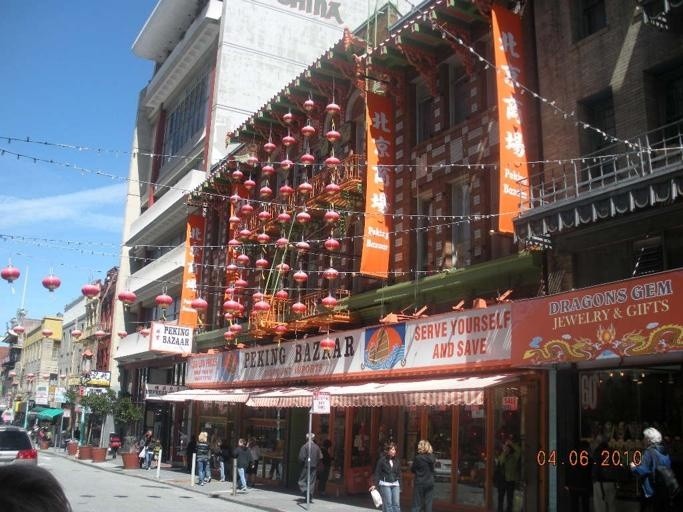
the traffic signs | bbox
[311,390,332,414]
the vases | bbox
[40,441,48,449]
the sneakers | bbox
[240,486,246,491]
[199,477,210,486]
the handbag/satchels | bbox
[371,489,383,507]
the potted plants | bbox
[65,386,82,456]
[79,392,100,460]
[112,396,142,469]
[90,392,116,463]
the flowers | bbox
[31,426,50,441]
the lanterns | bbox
[118,292,136,304]
[224,98,340,342]
[42,277,61,291]
[1,266,20,282]
[82,285,100,299]
[192,297,208,312]
[155,293,173,307]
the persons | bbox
[63,432,71,453]
[153,440,163,468]
[630,427,670,512]
[142,430,154,471]
[109,433,121,459]
[369,444,403,512]
[410,440,436,512]
[592,443,618,512]
[37,427,49,447]
[317,439,335,498]
[187,432,261,491]
[0,463,71,512]
[496,439,522,511]
[564,440,591,512]
[297,433,324,502]
[75,427,80,441]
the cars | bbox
[430,451,462,484]
[175,426,191,449]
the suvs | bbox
[0,424,37,467]
